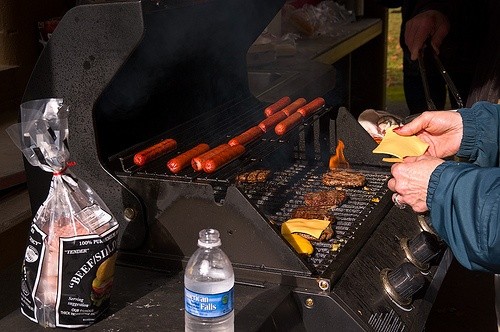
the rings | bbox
[393,193,407,210]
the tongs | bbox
[413,41,464,111]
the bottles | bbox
[183,229,234,332]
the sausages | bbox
[133,96,326,172]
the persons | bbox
[398,0,500,115]
[388,101,500,274]
